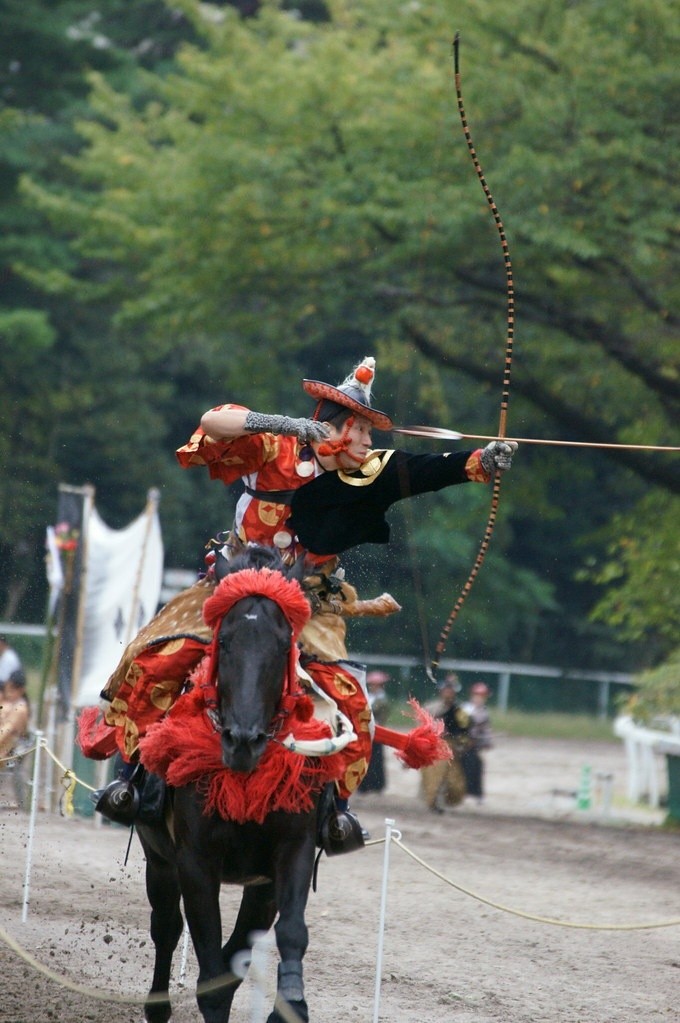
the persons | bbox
[0,634,31,757]
[358,671,495,815]
[93,356,518,839]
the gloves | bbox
[479,440,518,475]
[243,412,331,446]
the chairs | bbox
[0,727,40,807]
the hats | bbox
[303,357,393,431]
[367,671,390,685]
[472,683,493,697]
[438,675,462,692]
[9,671,25,683]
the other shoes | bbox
[361,828,370,841]
[91,790,106,806]
[434,803,446,813]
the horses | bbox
[128,550,340,1023]
